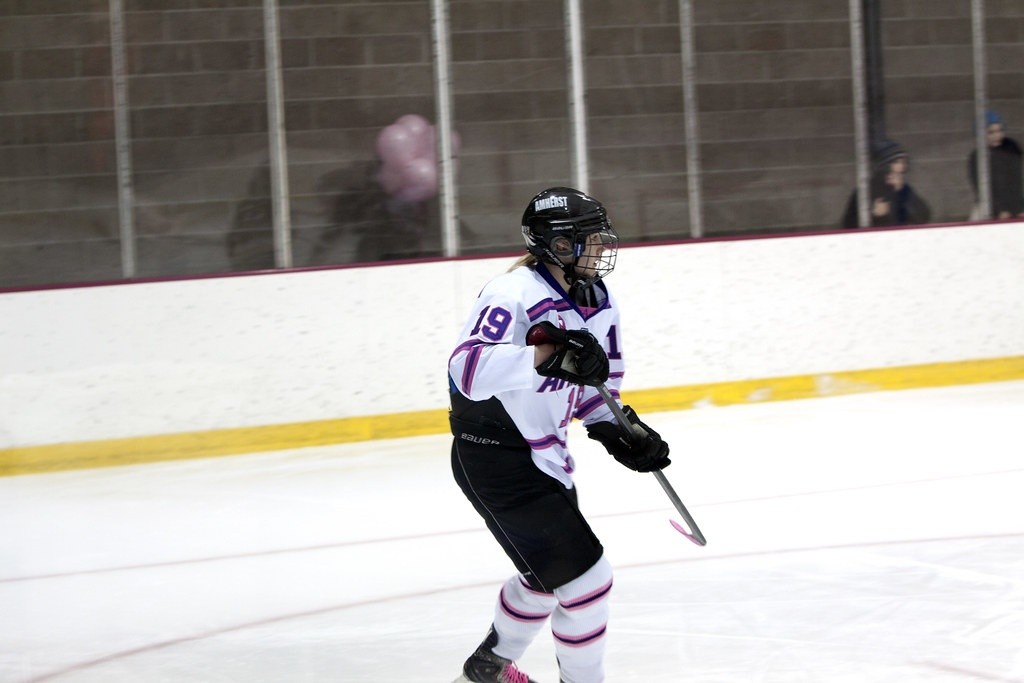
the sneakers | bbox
[451,622,538,683]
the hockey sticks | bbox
[527,322,706,548]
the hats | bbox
[871,142,908,175]
[972,112,1000,128]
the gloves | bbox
[525,320,609,388]
[584,405,671,473]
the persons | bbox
[840,139,929,230]
[443,187,671,683]
[968,111,1024,221]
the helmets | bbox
[521,187,620,289]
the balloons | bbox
[377,115,462,205]
